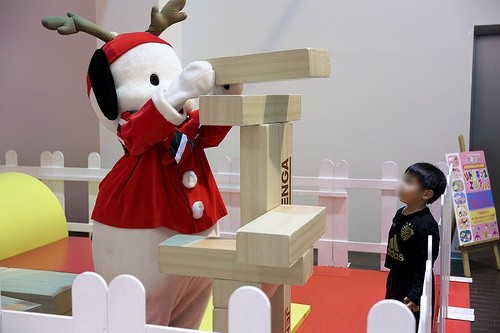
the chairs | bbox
[0,173,94,315]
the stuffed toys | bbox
[41,0,244,331]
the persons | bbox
[384,162,447,333]
